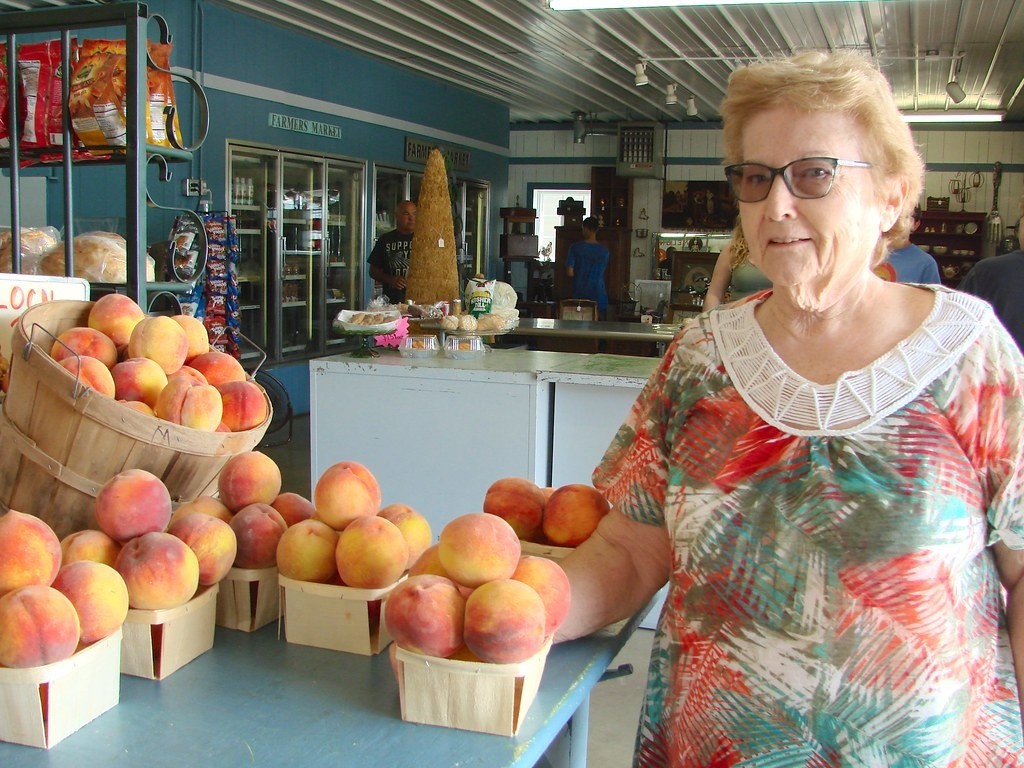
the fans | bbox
[246,369,295,449]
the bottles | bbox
[233,177,254,205]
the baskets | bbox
[0,540,575,751]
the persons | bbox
[551,49,1024,767]
[565,217,610,321]
[870,200,941,285]
[956,194,1024,353]
[367,199,416,304]
[661,246,691,290]
[703,212,772,313]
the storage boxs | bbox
[557,197,585,227]
[398,334,442,361]
[0,538,574,749]
[442,334,493,361]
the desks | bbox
[538,348,672,629]
[487,318,684,357]
[0,587,663,768]
[307,346,587,546]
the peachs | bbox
[275,461,432,627]
[483,476,612,548]
[51,293,267,433]
[0,449,316,725]
[384,513,571,665]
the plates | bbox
[965,222,977,234]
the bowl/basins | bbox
[933,246,947,254]
[915,245,929,253]
[953,250,975,255]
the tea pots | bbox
[942,264,959,278]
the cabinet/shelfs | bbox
[910,206,991,289]
[554,223,634,315]
[0,4,208,316]
[589,166,633,226]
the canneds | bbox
[452,299,461,316]
[440,301,450,316]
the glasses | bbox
[723,155,872,205]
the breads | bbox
[440,314,504,330]
[350,313,396,326]
[0,225,155,283]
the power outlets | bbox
[183,177,207,197]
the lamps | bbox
[665,81,678,106]
[943,61,967,102]
[633,58,651,86]
[685,92,699,117]
